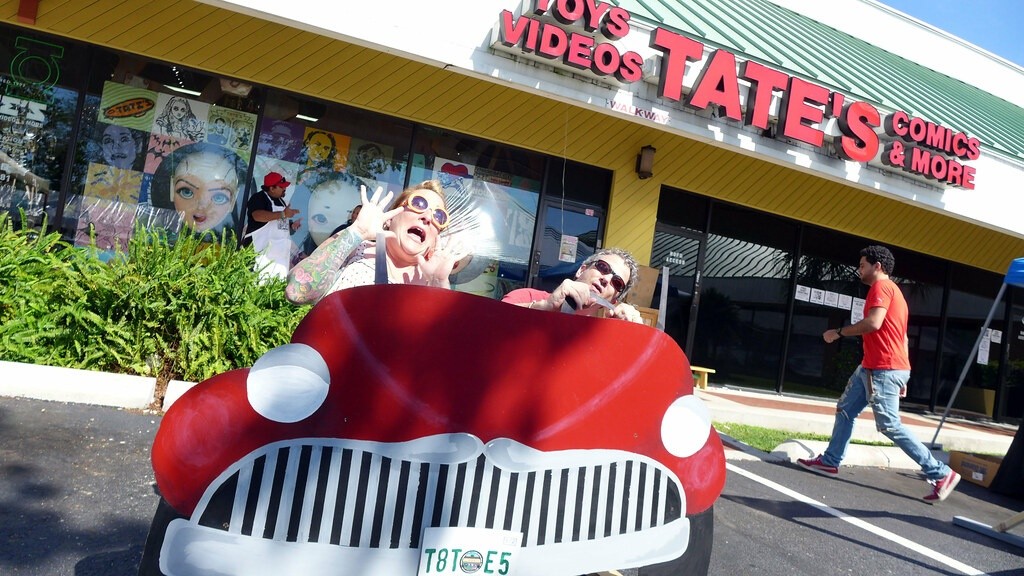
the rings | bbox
[622,309,626,314]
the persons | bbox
[330,204,362,238]
[502,247,644,324]
[242,171,302,286]
[797,246,961,502]
[285,180,474,305]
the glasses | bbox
[396,193,450,232]
[273,177,286,188]
[586,259,626,299]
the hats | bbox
[263,172,291,188]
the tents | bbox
[931,258,1024,447]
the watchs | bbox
[836,328,843,336]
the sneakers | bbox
[798,452,839,479]
[922,469,961,504]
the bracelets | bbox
[529,300,536,308]
[281,211,285,221]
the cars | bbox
[138,282,726,576]
